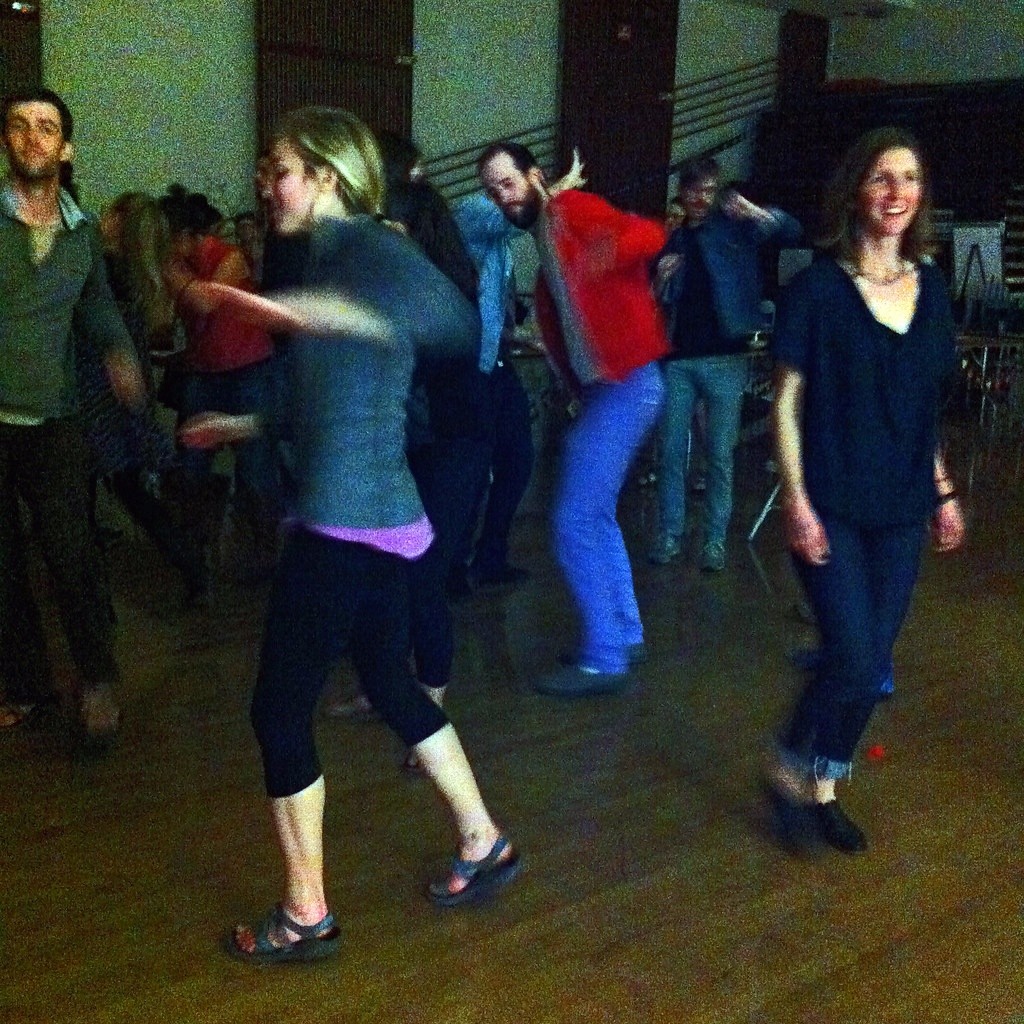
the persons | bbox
[186,106,519,963]
[100,132,588,599]
[479,141,666,695]
[0,88,149,738]
[648,156,803,574]
[762,124,963,855]
[668,197,687,228]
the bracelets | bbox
[937,489,959,506]
[933,475,952,484]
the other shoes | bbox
[476,564,530,586]
[763,775,867,851]
[402,753,422,775]
[535,642,648,697]
[78,683,120,734]
[324,687,380,721]
[1,702,37,728]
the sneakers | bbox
[649,534,683,564]
[700,539,725,572]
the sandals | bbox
[430,835,522,909]
[224,904,343,963]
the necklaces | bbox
[857,255,906,284]
